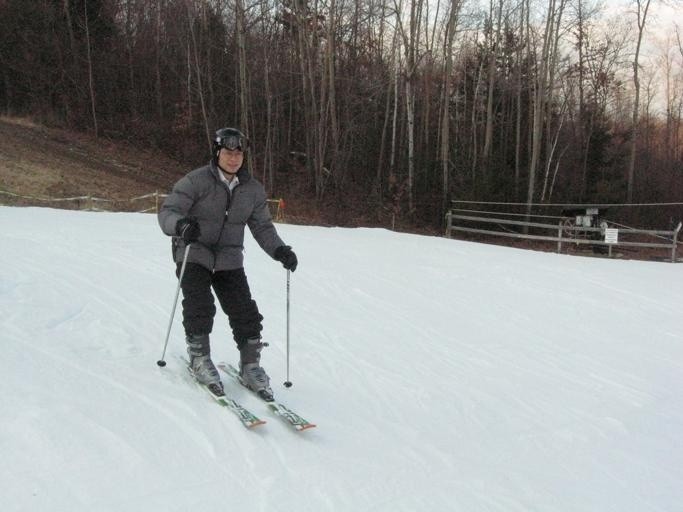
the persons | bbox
[156,126,299,395]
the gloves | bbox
[179,222,201,245]
[276,245,297,272]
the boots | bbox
[186,332,219,384]
[237,340,271,388]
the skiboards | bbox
[176,351,315,431]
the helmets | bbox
[213,127,250,152]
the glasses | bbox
[219,134,248,151]
